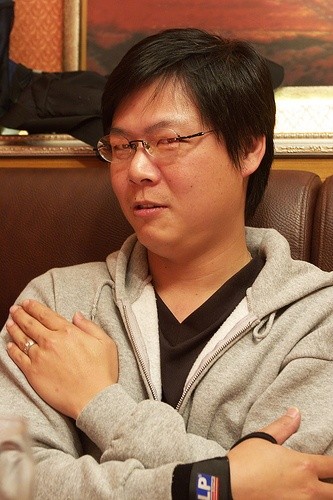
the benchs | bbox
[0,167,333,331]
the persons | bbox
[0,28,333,500]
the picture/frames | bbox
[62,0,333,131]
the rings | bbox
[22,340,37,355]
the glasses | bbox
[93,127,219,164]
